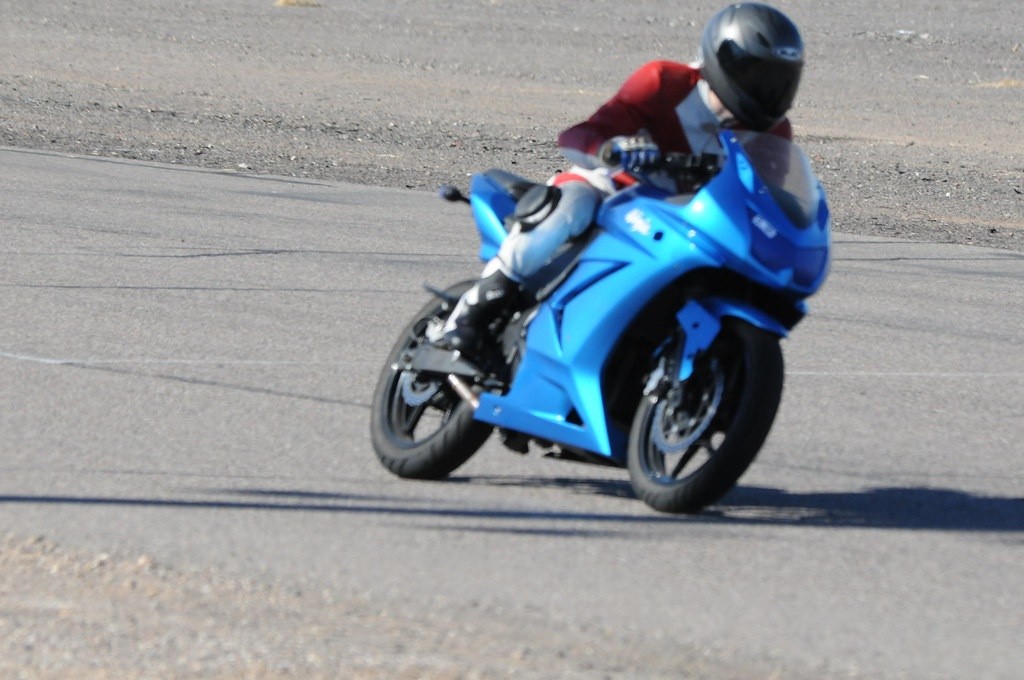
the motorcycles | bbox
[370,129,830,513]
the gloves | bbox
[599,129,660,173]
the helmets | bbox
[701,3,805,132]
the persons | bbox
[444,3,805,350]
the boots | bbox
[444,254,529,356]
